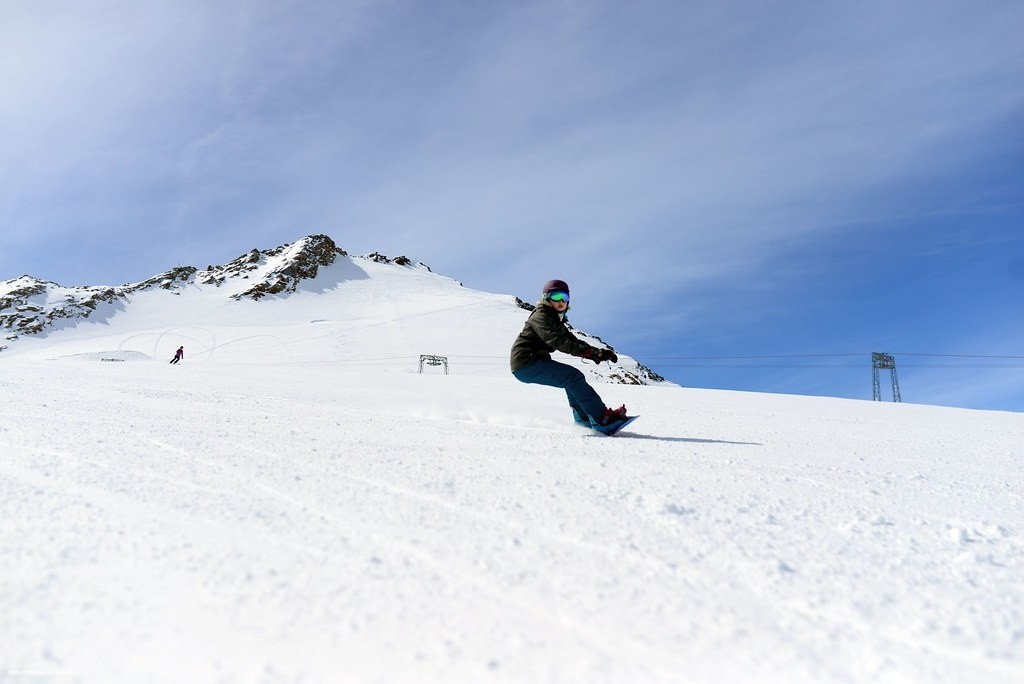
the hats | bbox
[543,280,568,294]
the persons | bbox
[170,346,183,364]
[510,280,628,432]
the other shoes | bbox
[582,406,625,426]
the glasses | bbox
[544,292,569,302]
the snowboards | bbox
[609,414,642,437]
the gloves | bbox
[578,341,617,364]
